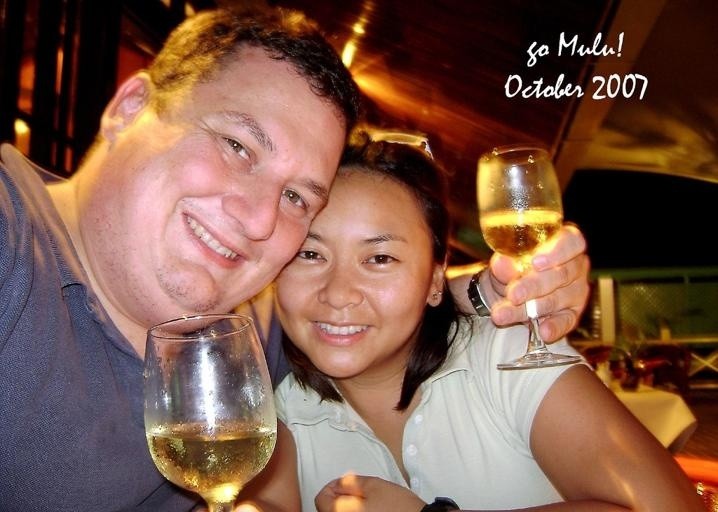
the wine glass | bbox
[476,145,581,371]
[141,314,276,512]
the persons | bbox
[194,140,712,512]
[0,0,593,512]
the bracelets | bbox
[420,497,459,512]
[466,271,491,316]
